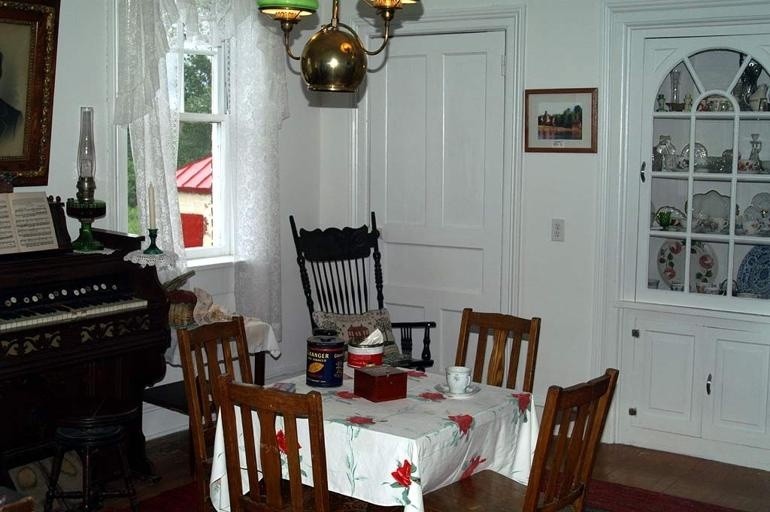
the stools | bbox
[42,397,142,511]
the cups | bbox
[446,365,472,393]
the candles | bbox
[147,182,156,230]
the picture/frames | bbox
[1,2,61,187]
[522,87,597,154]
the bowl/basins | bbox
[655,95,769,237]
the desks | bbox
[142,316,270,420]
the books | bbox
[1,190,60,254]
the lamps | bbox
[254,0,421,92]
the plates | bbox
[735,244,769,297]
[434,383,482,399]
[657,237,719,292]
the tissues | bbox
[348,327,385,368]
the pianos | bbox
[1,195,172,511]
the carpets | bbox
[588,477,738,511]
[129,479,215,512]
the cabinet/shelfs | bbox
[633,35,770,300]
[612,307,770,473]
